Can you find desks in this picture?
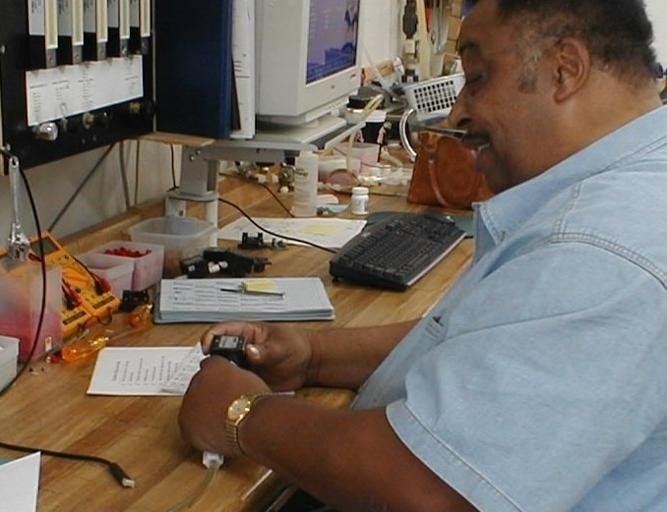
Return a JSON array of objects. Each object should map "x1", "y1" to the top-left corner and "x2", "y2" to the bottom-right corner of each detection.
[{"x1": 0, "y1": 164, "x2": 495, "y2": 512}]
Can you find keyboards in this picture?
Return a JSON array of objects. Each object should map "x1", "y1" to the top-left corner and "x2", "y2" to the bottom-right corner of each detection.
[{"x1": 329, "y1": 214, "x2": 468, "y2": 292}]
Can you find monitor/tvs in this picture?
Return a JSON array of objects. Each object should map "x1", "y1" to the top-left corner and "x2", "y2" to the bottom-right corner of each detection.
[{"x1": 254, "y1": 0, "x2": 362, "y2": 146}]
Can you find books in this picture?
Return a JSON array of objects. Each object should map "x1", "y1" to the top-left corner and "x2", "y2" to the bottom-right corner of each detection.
[
  {"x1": 153, "y1": 278, "x2": 335, "y2": 324},
  {"x1": 155, "y1": 0, "x2": 256, "y2": 139}
]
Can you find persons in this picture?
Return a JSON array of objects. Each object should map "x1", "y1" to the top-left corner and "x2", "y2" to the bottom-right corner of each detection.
[{"x1": 179, "y1": 1, "x2": 667, "y2": 511}]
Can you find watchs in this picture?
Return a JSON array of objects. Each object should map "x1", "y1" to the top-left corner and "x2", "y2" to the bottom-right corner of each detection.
[{"x1": 224, "y1": 390, "x2": 295, "y2": 458}]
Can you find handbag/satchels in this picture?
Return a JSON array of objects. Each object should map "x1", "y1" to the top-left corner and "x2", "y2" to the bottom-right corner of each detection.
[{"x1": 407, "y1": 125, "x2": 499, "y2": 210}]
